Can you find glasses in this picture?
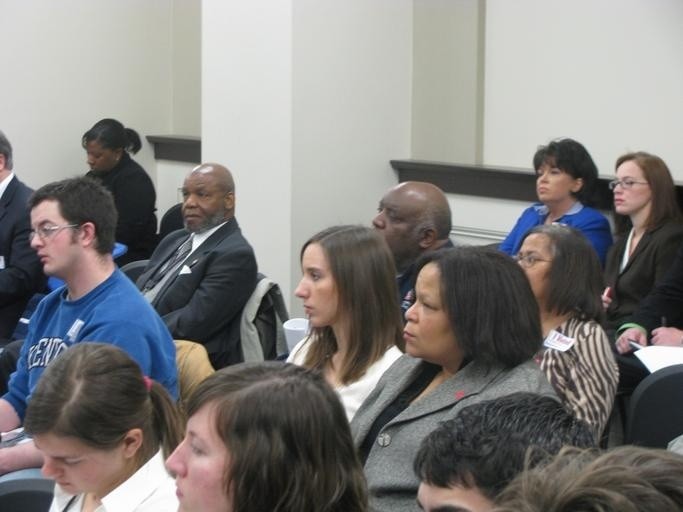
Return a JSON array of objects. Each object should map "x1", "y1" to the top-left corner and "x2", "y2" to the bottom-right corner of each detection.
[
  {"x1": 27, "y1": 224, "x2": 79, "y2": 242},
  {"x1": 512, "y1": 253, "x2": 551, "y2": 267},
  {"x1": 608, "y1": 179, "x2": 649, "y2": 190}
]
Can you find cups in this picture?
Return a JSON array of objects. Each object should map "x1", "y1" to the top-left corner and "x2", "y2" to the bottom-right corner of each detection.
[{"x1": 282, "y1": 317, "x2": 308, "y2": 354}]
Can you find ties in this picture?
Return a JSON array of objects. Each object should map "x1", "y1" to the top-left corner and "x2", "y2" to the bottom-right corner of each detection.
[{"x1": 143, "y1": 238, "x2": 192, "y2": 290}]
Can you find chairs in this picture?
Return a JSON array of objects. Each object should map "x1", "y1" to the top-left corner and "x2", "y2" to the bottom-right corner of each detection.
[
  {"x1": 620, "y1": 361, "x2": 682, "y2": 454},
  {"x1": 0, "y1": 465, "x2": 56, "y2": 512},
  {"x1": 113, "y1": 253, "x2": 282, "y2": 377},
  {"x1": 157, "y1": 201, "x2": 189, "y2": 240},
  {"x1": 585, "y1": 392, "x2": 626, "y2": 464}
]
